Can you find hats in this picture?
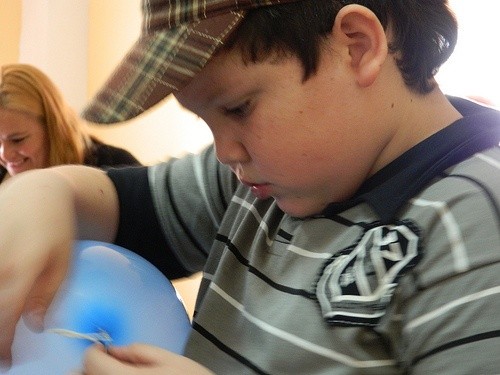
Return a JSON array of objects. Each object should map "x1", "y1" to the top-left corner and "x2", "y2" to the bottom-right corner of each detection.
[{"x1": 80, "y1": 0, "x2": 293, "y2": 124}]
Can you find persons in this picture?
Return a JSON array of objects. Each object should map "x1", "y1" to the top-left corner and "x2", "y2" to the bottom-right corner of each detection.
[
  {"x1": 0, "y1": 0, "x2": 500, "y2": 375},
  {"x1": 0, "y1": 63, "x2": 144, "y2": 185}
]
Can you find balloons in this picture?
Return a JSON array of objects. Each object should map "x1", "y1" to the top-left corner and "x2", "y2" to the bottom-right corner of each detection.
[{"x1": 0, "y1": 240, "x2": 191, "y2": 375}]
